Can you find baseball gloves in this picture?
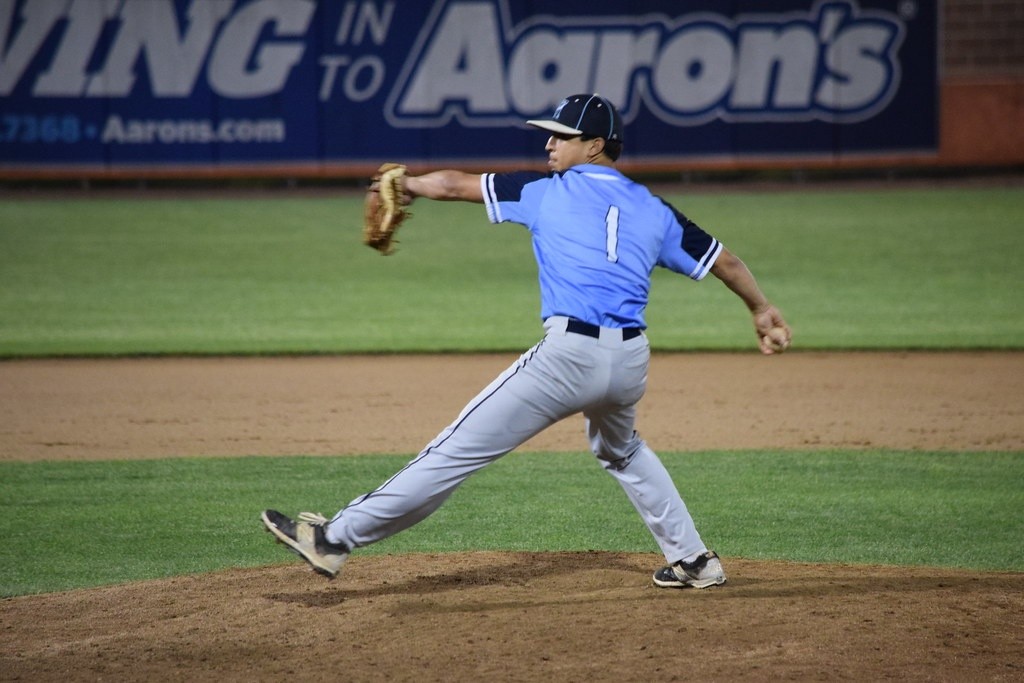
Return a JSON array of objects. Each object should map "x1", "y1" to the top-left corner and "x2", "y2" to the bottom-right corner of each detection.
[{"x1": 362, "y1": 161, "x2": 415, "y2": 257}]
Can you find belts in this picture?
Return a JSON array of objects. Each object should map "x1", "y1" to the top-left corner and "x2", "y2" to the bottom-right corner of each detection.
[{"x1": 565, "y1": 319, "x2": 641, "y2": 341}]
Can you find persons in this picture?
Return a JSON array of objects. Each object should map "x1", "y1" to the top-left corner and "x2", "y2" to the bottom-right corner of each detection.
[{"x1": 260, "y1": 92, "x2": 791, "y2": 589}]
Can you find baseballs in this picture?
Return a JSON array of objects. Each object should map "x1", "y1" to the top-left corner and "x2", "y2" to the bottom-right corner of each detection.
[{"x1": 763, "y1": 326, "x2": 789, "y2": 353}]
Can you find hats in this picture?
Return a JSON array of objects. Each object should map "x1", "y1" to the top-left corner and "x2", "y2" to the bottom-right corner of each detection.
[{"x1": 525, "y1": 93, "x2": 622, "y2": 143}]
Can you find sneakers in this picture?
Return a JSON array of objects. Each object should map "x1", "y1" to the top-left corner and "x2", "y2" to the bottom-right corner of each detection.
[
  {"x1": 653, "y1": 549, "x2": 727, "y2": 588},
  {"x1": 260, "y1": 508, "x2": 351, "y2": 578}
]
